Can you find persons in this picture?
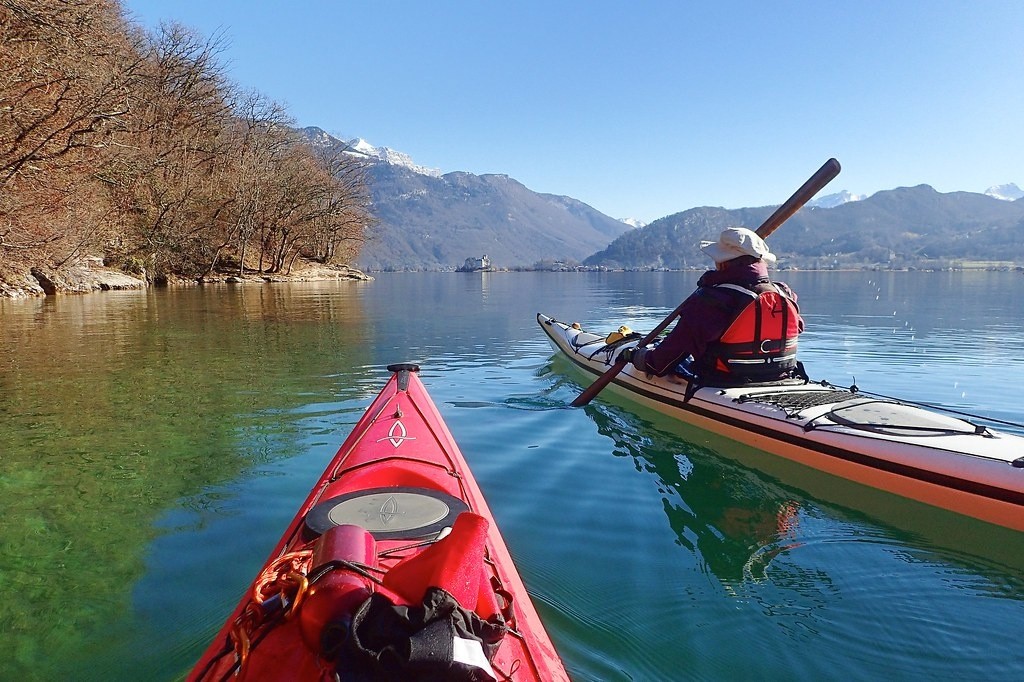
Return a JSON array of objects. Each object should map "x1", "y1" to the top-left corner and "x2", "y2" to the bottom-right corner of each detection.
[{"x1": 614, "y1": 227, "x2": 806, "y2": 388}]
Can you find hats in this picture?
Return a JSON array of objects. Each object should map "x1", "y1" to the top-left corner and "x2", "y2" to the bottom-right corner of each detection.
[{"x1": 700, "y1": 228, "x2": 777, "y2": 265}]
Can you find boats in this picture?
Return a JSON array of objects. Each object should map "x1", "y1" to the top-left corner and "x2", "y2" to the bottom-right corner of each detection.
[
  {"x1": 536, "y1": 312, "x2": 1024, "y2": 531},
  {"x1": 184, "y1": 364, "x2": 570, "y2": 682}
]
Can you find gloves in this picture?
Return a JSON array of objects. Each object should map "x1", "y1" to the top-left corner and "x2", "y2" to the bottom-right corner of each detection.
[{"x1": 615, "y1": 346, "x2": 640, "y2": 364}]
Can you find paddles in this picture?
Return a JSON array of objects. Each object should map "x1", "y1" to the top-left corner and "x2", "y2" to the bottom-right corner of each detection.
[{"x1": 569, "y1": 157, "x2": 843, "y2": 408}]
